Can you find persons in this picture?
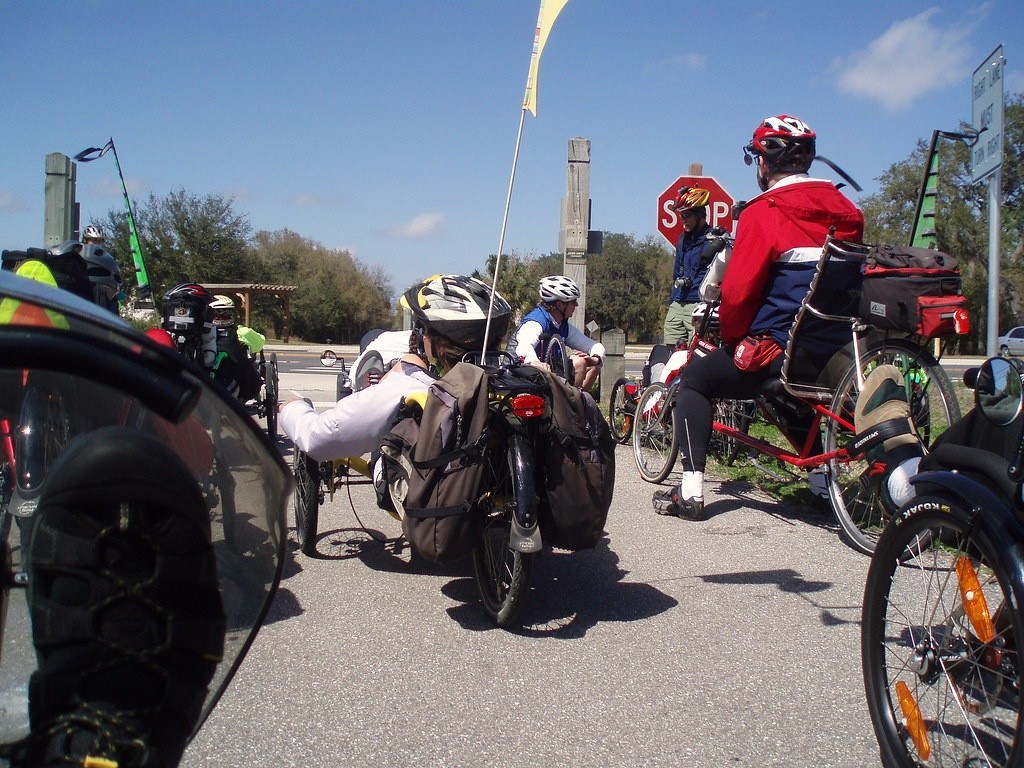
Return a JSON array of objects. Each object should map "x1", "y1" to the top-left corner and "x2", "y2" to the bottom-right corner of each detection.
[
  {"x1": 21, "y1": 226, "x2": 261, "y2": 542},
  {"x1": 0, "y1": 429, "x2": 226, "y2": 768},
  {"x1": 654, "y1": 116, "x2": 866, "y2": 522},
  {"x1": 854, "y1": 364, "x2": 1024, "y2": 548},
  {"x1": 278, "y1": 274, "x2": 510, "y2": 525},
  {"x1": 664, "y1": 187, "x2": 725, "y2": 360},
  {"x1": 503, "y1": 275, "x2": 606, "y2": 399}
]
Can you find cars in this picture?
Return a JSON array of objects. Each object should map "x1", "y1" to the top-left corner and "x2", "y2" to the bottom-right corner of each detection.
[{"x1": 997, "y1": 326, "x2": 1024, "y2": 357}]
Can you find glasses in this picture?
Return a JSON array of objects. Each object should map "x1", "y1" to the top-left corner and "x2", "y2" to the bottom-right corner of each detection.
[
  {"x1": 681, "y1": 211, "x2": 698, "y2": 219},
  {"x1": 754, "y1": 154, "x2": 760, "y2": 165},
  {"x1": 89, "y1": 237, "x2": 103, "y2": 245},
  {"x1": 566, "y1": 298, "x2": 577, "y2": 304}
]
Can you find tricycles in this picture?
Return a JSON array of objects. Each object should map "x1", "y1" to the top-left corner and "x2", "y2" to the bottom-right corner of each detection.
[
  {"x1": 0, "y1": 271, "x2": 298, "y2": 768},
  {"x1": 610, "y1": 225, "x2": 963, "y2": 563},
  {"x1": 861, "y1": 358, "x2": 1024, "y2": 768},
  {"x1": 292, "y1": 350, "x2": 550, "y2": 628}
]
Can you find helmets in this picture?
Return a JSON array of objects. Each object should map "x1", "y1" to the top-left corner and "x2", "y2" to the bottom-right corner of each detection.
[
  {"x1": 673, "y1": 186, "x2": 710, "y2": 211},
  {"x1": 539, "y1": 276, "x2": 581, "y2": 303},
  {"x1": 398, "y1": 273, "x2": 513, "y2": 352},
  {"x1": 162, "y1": 283, "x2": 215, "y2": 305},
  {"x1": 83, "y1": 224, "x2": 106, "y2": 240},
  {"x1": 209, "y1": 295, "x2": 234, "y2": 311},
  {"x1": 748, "y1": 114, "x2": 816, "y2": 166}
]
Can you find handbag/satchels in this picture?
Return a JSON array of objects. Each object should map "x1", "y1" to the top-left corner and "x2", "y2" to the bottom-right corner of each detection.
[
  {"x1": 400, "y1": 358, "x2": 616, "y2": 558},
  {"x1": 859, "y1": 245, "x2": 960, "y2": 335}
]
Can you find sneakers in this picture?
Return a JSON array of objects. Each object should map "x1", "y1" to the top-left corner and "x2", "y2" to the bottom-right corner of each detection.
[
  {"x1": 21, "y1": 427, "x2": 226, "y2": 768},
  {"x1": 653, "y1": 483, "x2": 703, "y2": 521},
  {"x1": 373, "y1": 456, "x2": 392, "y2": 510},
  {"x1": 844, "y1": 364, "x2": 929, "y2": 517}
]
